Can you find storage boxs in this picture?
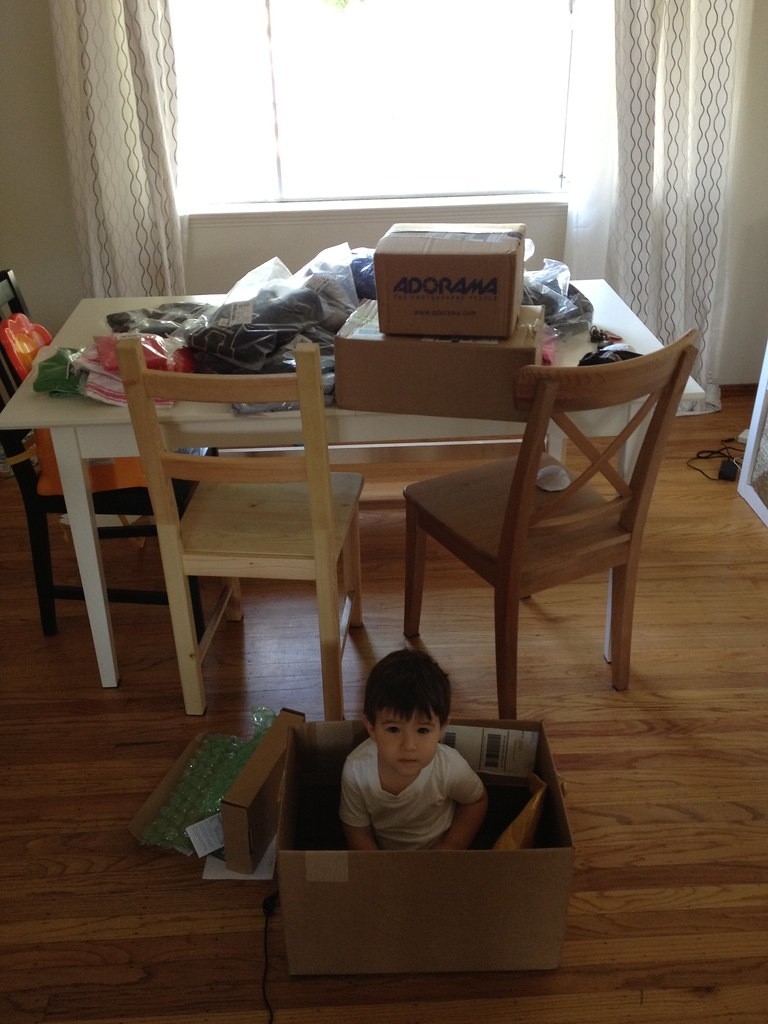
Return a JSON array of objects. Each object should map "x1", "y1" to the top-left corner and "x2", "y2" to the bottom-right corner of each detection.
[
  {"x1": 124, "y1": 708, "x2": 303, "y2": 876},
  {"x1": 371, "y1": 222, "x2": 528, "y2": 337},
  {"x1": 333, "y1": 295, "x2": 550, "y2": 423},
  {"x1": 273, "y1": 712, "x2": 580, "y2": 979}
]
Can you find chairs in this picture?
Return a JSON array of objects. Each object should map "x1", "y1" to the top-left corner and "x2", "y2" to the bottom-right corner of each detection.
[
  {"x1": 401, "y1": 328, "x2": 694, "y2": 723},
  {"x1": 116, "y1": 333, "x2": 362, "y2": 718},
  {"x1": 0, "y1": 269, "x2": 216, "y2": 636}
]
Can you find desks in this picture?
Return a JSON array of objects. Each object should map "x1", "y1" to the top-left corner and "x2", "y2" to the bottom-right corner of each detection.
[
  {"x1": 1, "y1": 279, "x2": 706, "y2": 688},
  {"x1": 0, "y1": 277, "x2": 708, "y2": 690}
]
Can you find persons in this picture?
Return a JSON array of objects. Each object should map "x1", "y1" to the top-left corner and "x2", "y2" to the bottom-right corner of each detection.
[{"x1": 335, "y1": 649, "x2": 489, "y2": 850}]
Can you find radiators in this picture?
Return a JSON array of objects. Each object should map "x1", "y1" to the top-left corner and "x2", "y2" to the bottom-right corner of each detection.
[{"x1": 183, "y1": 199, "x2": 568, "y2": 304}]
